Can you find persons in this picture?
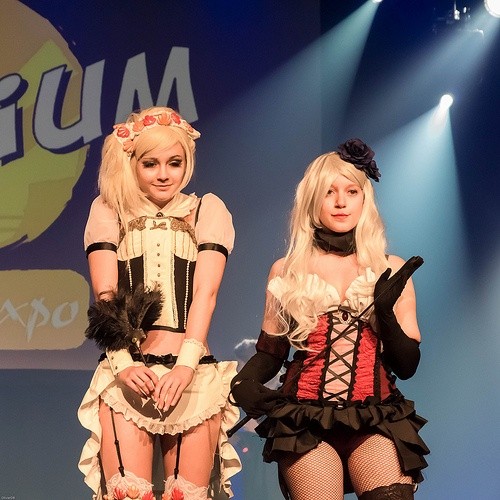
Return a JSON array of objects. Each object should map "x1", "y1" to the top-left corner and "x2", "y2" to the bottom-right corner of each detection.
[
  {"x1": 78, "y1": 106, "x2": 242, "y2": 500},
  {"x1": 227, "y1": 138, "x2": 430, "y2": 500}
]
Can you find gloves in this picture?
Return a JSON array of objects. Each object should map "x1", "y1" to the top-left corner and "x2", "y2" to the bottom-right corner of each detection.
[
  {"x1": 372, "y1": 255, "x2": 421, "y2": 382},
  {"x1": 229, "y1": 330, "x2": 287, "y2": 420}
]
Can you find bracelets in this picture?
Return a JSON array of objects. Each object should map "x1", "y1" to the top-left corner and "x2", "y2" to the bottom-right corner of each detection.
[
  {"x1": 175, "y1": 339, "x2": 206, "y2": 370},
  {"x1": 104, "y1": 347, "x2": 135, "y2": 374}
]
